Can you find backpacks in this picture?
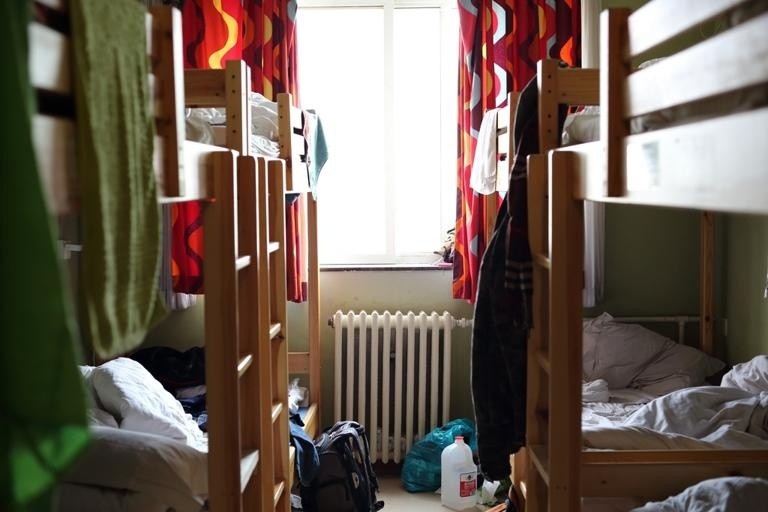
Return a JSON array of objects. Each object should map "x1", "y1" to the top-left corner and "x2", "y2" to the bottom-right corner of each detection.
[{"x1": 301, "y1": 421, "x2": 384, "y2": 512}]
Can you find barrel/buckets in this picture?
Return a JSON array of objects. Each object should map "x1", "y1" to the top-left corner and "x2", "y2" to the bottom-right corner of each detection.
[{"x1": 434, "y1": 431, "x2": 483, "y2": 508}]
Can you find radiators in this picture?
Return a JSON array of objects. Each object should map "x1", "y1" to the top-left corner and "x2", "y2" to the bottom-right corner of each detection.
[{"x1": 330, "y1": 308, "x2": 472, "y2": 468}]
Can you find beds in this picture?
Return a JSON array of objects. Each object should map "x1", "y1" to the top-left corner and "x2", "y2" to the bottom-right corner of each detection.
[
  {"x1": 527, "y1": 1, "x2": 767, "y2": 512},
  {"x1": 475, "y1": 57, "x2": 767, "y2": 512},
  {"x1": 0, "y1": 0, "x2": 265, "y2": 512},
  {"x1": 54, "y1": 56, "x2": 329, "y2": 512}
]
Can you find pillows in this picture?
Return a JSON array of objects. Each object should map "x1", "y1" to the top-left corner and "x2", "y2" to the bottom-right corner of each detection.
[
  {"x1": 579, "y1": 309, "x2": 615, "y2": 379},
  {"x1": 581, "y1": 313, "x2": 724, "y2": 401},
  {"x1": 59, "y1": 423, "x2": 208, "y2": 497}
]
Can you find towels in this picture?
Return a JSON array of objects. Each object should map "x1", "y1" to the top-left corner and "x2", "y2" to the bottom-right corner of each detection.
[
  {"x1": 469, "y1": 107, "x2": 501, "y2": 195},
  {"x1": 301, "y1": 107, "x2": 329, "y2": 201}
]
